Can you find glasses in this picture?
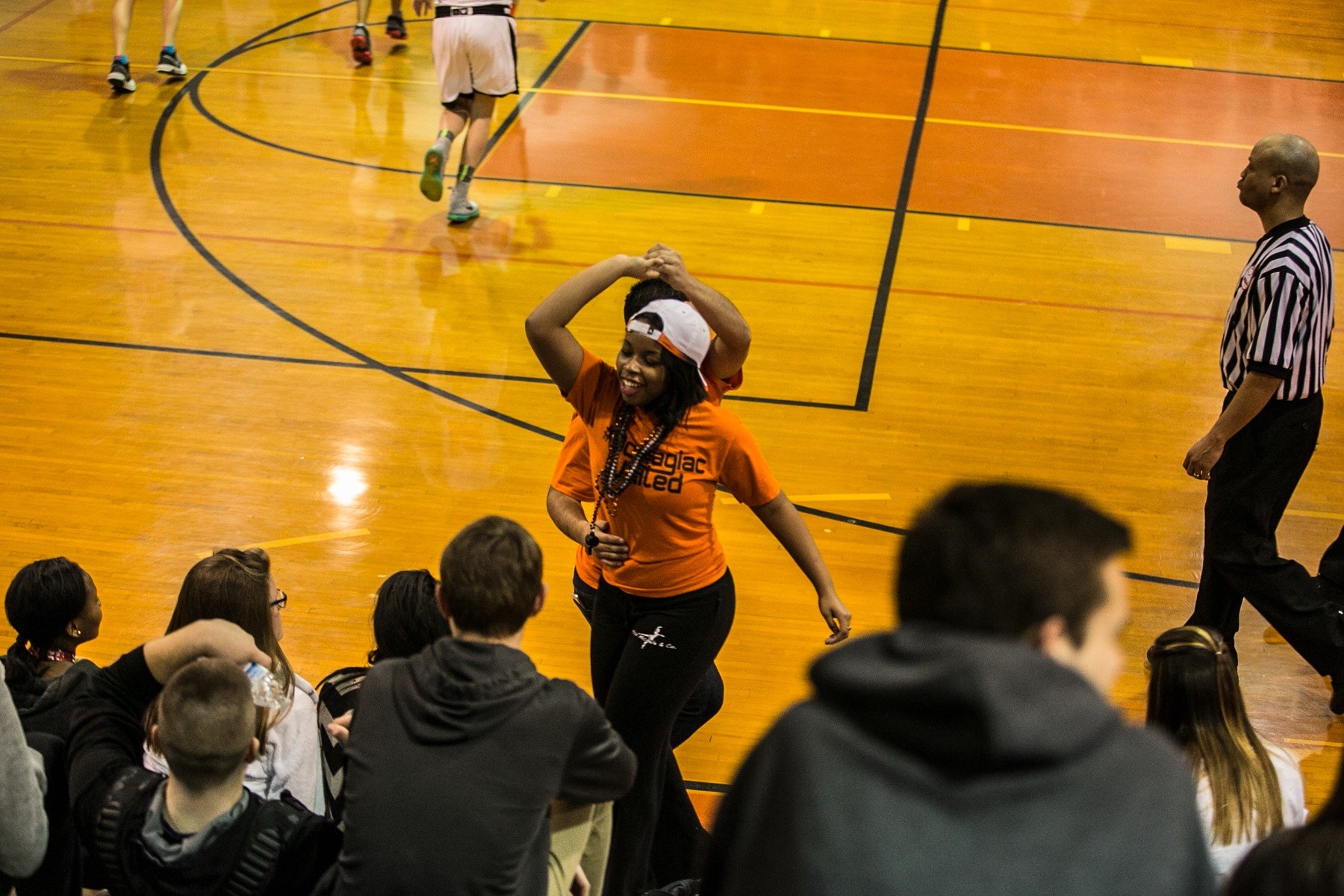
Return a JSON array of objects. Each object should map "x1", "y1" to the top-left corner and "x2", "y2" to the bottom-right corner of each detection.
[{"x1": 270, "y1": 588, "x2": 287, "y2": 609}]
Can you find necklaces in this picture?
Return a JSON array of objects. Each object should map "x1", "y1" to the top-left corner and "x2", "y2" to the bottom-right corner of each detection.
[{"x1": 584, "y1": 392, "x2": 679, "y2": 555}]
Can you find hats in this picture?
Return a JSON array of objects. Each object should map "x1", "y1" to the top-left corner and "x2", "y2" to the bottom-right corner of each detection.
[{"x1": 627, "y1": 298, "x2": 713, "y2": 387}]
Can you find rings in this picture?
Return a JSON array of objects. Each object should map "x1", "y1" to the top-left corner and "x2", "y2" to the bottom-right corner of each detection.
[{"x1": 847, "y1": 626, "x2": 851, "y2": 630}]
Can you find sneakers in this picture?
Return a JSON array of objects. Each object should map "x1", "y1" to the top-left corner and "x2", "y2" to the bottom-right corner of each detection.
[
  {"x1": 105, "y1": 60, "x2": 137, "y2": 92},
  {"x1": 447, "y1": 197, "x2": 479, "y2": 222},
  {"x1": 420, "y1": 140, "x2": 449, "y2": 201},
  {"x1": 155, "y1": 51, "x2": 188, "y2": 75}
]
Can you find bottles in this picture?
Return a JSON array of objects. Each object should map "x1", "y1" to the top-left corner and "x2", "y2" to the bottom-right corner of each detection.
[{"x1": 241, "y1": 661, "x2": 291, "y2": 711}]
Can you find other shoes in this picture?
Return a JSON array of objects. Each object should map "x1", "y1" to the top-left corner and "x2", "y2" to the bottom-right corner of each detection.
[
  {"x1": 386, "y1": 15, "x2": 408, "y2": 40},
  {"x1": 1328, "y1": 611, "x2": 1344, "y2": 715},
  {"x1": 351, "y1": 23, "x2": 373, "y2": 65},
  {"x1": 1264, "y1": 625, "x2": 1288, "y2": 645}
]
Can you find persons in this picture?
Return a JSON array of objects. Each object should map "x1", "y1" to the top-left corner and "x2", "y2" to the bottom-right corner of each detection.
[
  {"x1": 1146, "y1": 134, "x2": 1344, "y2": 714},
  {"x1": 1226, "y1": 525, "x2": 1344, "y2": 896},
  {"x1": 0, "y1": 514, "x2": 634, "y2": 896},
  {"x1": 353, "y1": 0, "x2": 553, "y2": 219},
  {"x1": 1142, "y1": 625, "x2": 1307, "y2": 888},
  {"x1": 107, "y1": 0, "x2": 188, "y2": 94},
  {"x1": 693, "y1": 476, "x2": 1217, "y2": 896},
  {"x1": 544, "y1": 242, "x2": 751, "y2": 754},
  {"x1": 525, "y1": 252, "x2": 852, "y2": 896}
]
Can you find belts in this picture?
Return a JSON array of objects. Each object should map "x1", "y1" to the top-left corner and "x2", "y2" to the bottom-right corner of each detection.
[{"x1": 435, "y1": 6, "x2": 512, "y2": 16}]
